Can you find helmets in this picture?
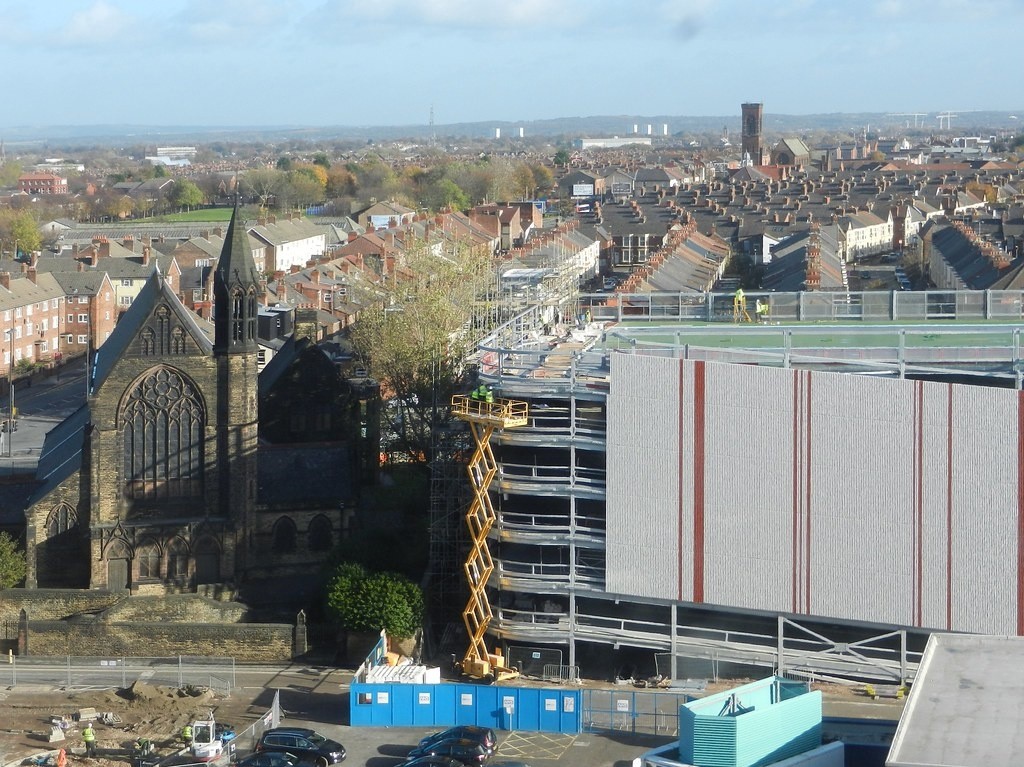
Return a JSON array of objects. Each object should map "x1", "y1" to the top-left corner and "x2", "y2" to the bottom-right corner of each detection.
[{"x1": 88, "y1": 723, "x2": 92, "y2": 726}]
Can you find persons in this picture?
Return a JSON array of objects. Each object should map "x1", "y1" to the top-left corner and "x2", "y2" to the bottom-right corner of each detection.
[
  {"x1": 537, "y1": 314, "x2": 549, "y2": 336},
  {"x1": 136, "y1": 737, "x2": 150, "y2": 755},
  {"x1": 754, "y1": 299, "x2": 762, "y2": 323},
  {"x1": 182, "y1": 723, "x2": 192, "y2": 747},
  {"x1": 82, "y1": 722, "x2": 97, "y2": 758},
  {"x1": 736, "y1": 288, "x2": 744, "y2": 309},
  {"x1": 585, "y1": 310, "x2": 591, "y2": 324},
  {"x1": 472, "y1": 383, "x2": 494, "y2": 414}
]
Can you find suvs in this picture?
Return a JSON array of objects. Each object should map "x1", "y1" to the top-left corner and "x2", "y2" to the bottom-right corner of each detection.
[
  {"x1": 407, "y1": 736, "x2": 491, "y2": 767},
  {"x1": 255, "y1": 726, "x2": 347, "y2": 765},
  {"x1": 417, "y1": 725, "x2": 499, "y2": 757}
]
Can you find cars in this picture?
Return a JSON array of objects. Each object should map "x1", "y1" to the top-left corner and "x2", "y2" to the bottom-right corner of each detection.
[
  {"x1": 386, "y1": 392, "x2": 419, "y2": 410},
  {"x1": 231, "y1": 747, "x2": 328, "y2": 767},
  {"x1": 392, "y1": 754, "x2": 465, "y2": 767},
  {"x1": 379, "y1": 435, "x2": 472, "y2": 469}
]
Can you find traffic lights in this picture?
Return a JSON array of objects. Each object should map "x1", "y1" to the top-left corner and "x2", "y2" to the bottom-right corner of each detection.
[
  {"x1": 2, "y1": 420, "x2": 9, "y2": 433},
  {"x1": 13, "y1": 420, "x2": 18, "y2": 431}
]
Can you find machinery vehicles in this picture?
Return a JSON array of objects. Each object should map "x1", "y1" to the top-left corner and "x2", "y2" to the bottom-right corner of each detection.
[
  {"x1": 449, "y1": 393, "x2": 530, "y2": 684},
  {"x1": 192, "y1": 710, "x2": 225, "y2": 763}
]
